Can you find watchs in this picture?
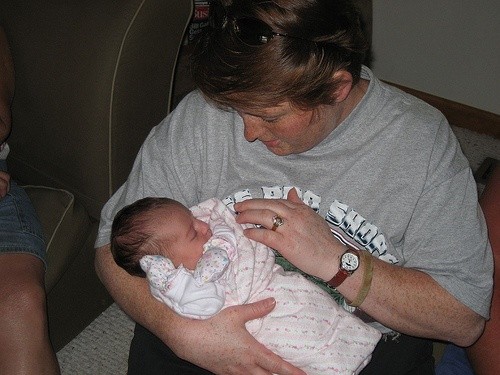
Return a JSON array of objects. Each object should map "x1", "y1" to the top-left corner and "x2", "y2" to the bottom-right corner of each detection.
[{"x1": 325, "y1": 243, "x2": 361, "y2": 291}]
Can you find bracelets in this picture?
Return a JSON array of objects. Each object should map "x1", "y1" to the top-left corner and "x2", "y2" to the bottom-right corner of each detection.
[{"x1": 345, "y1": 249, "x2": 374, "y2": 308}]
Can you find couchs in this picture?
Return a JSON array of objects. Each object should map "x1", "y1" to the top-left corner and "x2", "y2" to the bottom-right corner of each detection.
[{"x1": 0, "y1": 0, "x2": 194, "y2": 353}]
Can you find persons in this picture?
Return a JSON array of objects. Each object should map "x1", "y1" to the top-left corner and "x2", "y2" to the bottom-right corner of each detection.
[
  {"x1": 92, "y1": 1, "x2": 493, "y2": 375},
  {"x1": 1, "y1": 39, "x2": 60, "y2": 375}
]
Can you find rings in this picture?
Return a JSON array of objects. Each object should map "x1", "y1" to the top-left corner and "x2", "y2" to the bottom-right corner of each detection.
[{"x1": 271, "y1": 214, "x2": 283, "y2": 230}]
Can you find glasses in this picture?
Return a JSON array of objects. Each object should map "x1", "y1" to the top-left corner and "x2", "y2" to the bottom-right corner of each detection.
[{"x1": 209, "y1": 2, "x2": 297, "y2": 45}]
